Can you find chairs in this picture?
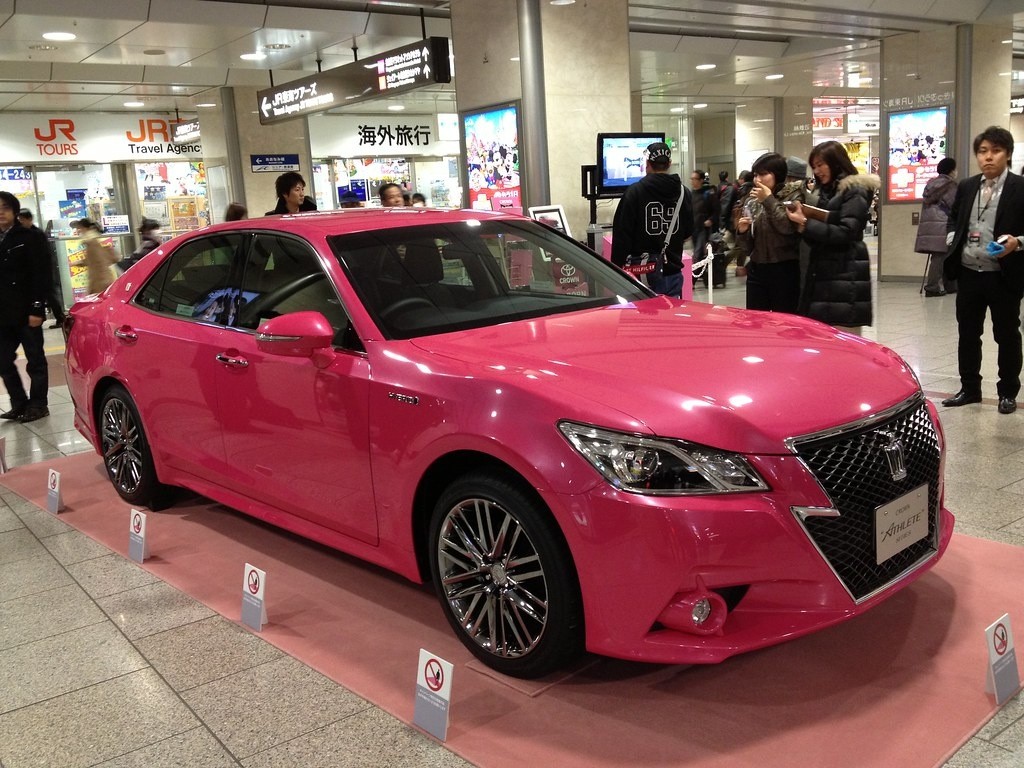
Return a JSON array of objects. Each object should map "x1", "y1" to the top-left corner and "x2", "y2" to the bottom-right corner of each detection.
[{"x1": 405, "y1": 241, "x2": 455, "y2": 311}]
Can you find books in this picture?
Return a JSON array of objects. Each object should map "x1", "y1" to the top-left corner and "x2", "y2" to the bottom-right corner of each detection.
[{"x1": 801, "y1": 204, "x2": 829, "y2": 223}]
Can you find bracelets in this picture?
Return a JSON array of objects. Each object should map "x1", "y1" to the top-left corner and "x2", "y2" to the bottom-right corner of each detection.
[{"x1": 798, "y1": 218, "x2": 807, "y2": 232}]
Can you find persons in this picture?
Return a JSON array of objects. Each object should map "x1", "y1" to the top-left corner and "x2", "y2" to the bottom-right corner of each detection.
[
  {"x1": 339, "y1": 190, "x2": 360, "y2": 208},
  {"x1": 17, "y1": 208, "x2": 66, "y2": 329},
  {"x1": 714, "y1": 171, "x2": 753, "y2": 277},
  {"x1": 785, "y1": 140, "x2": 882, "y2": 338},
  {"x1": 215, "y1": 203, "x2": 249, "y2": 264},
  {"x1": 610, "y1": 143, "x2": 694, "y2": 300},
  {"x1": 733, "y1": 153, "x2": 806, "y2": 313},
  {"x1": 0, "y1": 190, "x2": 53, "y2": 423},
  {"x1": 689, "y1": 169, "x2": 708, "y2": 290},
  {"x1": 116, "y1": 219, "x2": 166, "y2": 271},
  {"x1": 941, "y1": 127, "x2": 1024, "y2": 414},
  {"x1": 254, "y1": 171, "x2": 317, "y2": 271},
  {"x1": 378, "y1": 183, "x2": 445, "y2": 285},
  {"x1": 639, "y1": 149, "x2": 650, "y2": 177},
  {"x1": 914, "y1": 157, "x2": 958, "y2": 298}
]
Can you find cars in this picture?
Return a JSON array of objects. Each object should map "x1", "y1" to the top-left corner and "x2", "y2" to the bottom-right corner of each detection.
[{"x1": 61, "y1": 207, "x2": 955, "y2": 680}]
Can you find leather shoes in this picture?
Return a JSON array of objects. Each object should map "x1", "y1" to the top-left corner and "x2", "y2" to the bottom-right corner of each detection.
[
  {"x1": 998, "y1": 396, "x2": 1017, "y2": 414},
  {"x1": 941, "y1": 389, "x2": 982, "y2": 406}
]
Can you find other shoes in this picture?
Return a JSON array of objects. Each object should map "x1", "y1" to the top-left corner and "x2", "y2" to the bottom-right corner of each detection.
[
  {"x1": 713, "y1": 283, "x2": 724, "y2": 289},
  {"x1": 736, "y1": 266, "x2": 745, "y2": 276},
  {"x1": 16, "y1": 406, "x2": 50, "y2": 423},
  {"x1": 946, "y1": 287, "x2": 957, "y2": 294},
  {"x1": 924, "y1": 288, "x2": 947, "y2": 297},
  {"x1": 0, "y1": 407, "x2": 27, "y2": 419},
  {"x1": 49, "y1": 317, "x2": 66, "y2": 329}
]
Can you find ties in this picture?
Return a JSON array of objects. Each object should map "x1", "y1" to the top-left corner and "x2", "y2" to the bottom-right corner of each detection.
[{"x1": 980, "y1": 178, "x2": 995, "y2": 204}]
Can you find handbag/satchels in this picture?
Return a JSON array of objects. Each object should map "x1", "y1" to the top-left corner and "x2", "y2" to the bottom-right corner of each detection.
[{"x1": 623, "y1": 253, "x2": 664, "y2": 287}]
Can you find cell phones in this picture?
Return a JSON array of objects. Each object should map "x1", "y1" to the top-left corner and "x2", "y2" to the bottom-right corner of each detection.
[
  {"x1": 783, "y1": 201, "x2": 795, "y2": 211},
  {"x1": 997, "y1": 236, "x2": 1008, "y2": 245}
]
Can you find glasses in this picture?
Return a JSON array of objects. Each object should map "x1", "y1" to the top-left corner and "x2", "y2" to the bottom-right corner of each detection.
[{"x1": 688, "y1": 177, "x2": 699, "y2": 182}]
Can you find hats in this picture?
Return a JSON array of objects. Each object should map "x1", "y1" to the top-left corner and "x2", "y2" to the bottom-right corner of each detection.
[
  {"x1": 937, "y1": 158, "x2": 956, "y2": 175},
  {"x1": 784, "y1": 155, "x2": 808, "y2": 179}
]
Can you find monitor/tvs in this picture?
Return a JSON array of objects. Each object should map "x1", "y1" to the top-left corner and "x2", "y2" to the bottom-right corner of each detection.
[{"x1": 596, "y1": 132, "x2": 666, "y2": 193}]
